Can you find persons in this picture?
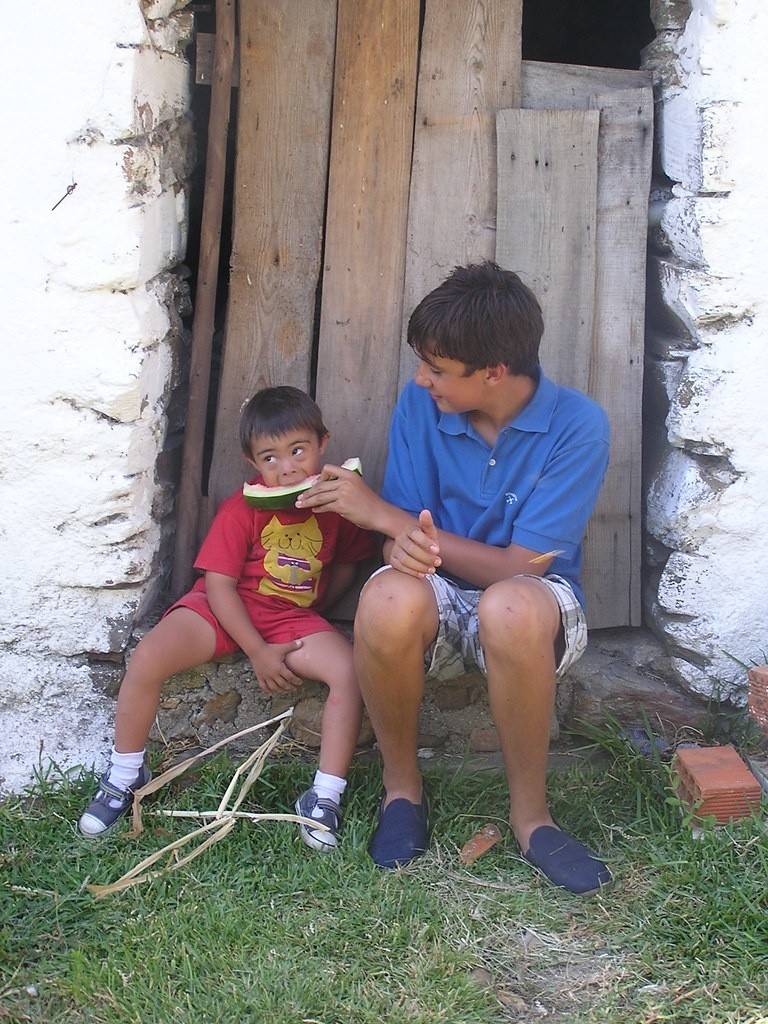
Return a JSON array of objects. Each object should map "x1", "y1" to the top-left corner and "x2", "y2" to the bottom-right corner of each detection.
[
  {"x1": 79, "y1": 384, "x2": 363, "y2": 853},
  {"x1": 295, "y1": 256, "x2": 614, "y2": 897}
]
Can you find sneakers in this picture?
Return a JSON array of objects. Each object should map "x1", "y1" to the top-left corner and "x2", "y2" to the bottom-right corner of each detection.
[
  {"x1": 79, "y1": 764, "x2": 152, "y2": 837},
  {"x1": 294, "y1": 787, "x2": 342, "y2": 852}
]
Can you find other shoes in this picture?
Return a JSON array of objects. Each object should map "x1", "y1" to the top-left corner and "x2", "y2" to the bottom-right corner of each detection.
[
  {"x1": 368, "y1": 785, "x2": 430, "y2": 870},
  {"x1": 508, "y1": 814, "x2": 614, "y2": 896}
]
